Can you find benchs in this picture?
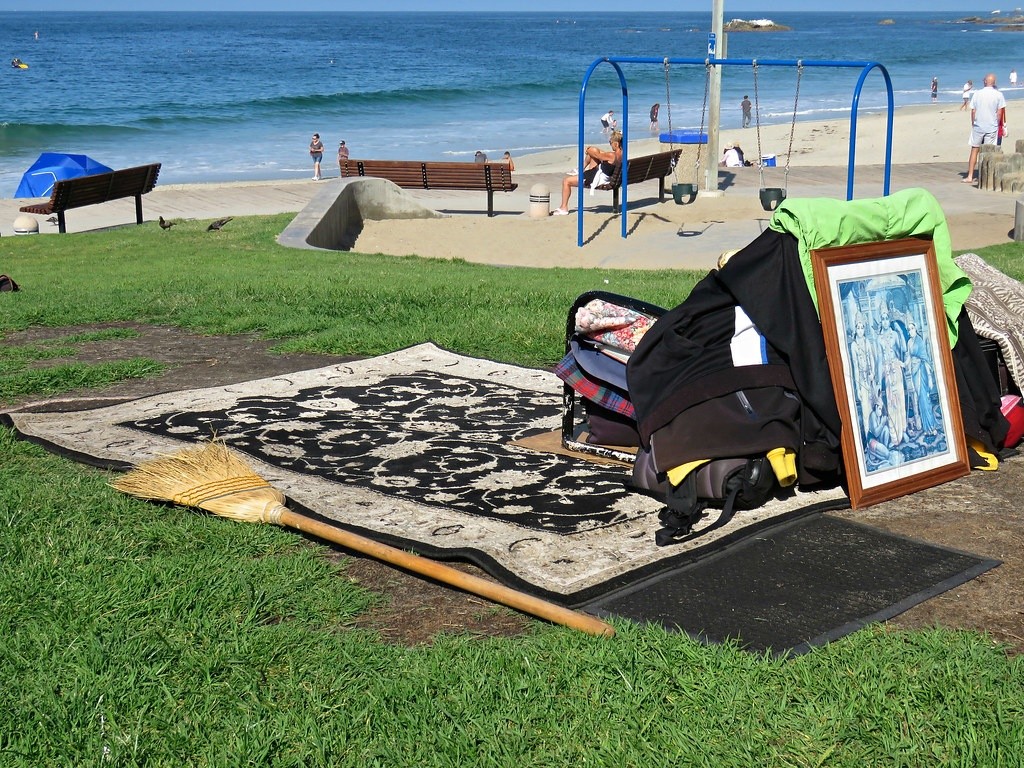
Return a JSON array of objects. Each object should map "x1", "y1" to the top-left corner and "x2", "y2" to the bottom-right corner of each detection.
[
  {"x1": 596, "y1": 149, "x2": 683, "y2": 214},
  {"x1": 339, "y1": 158, "x2": 519, "y2": 217},
  {"x1": 19, "y1": 162, "x2": 162, "y2": 233}
]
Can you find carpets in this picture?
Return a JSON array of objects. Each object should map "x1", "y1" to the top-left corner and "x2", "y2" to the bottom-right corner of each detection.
[
  {"x1": 0, "y1": 338, "x2": 849, "y2": 611},
  {"x1": 585, "y1": 512, "x2": 1002, "y2": 657}
]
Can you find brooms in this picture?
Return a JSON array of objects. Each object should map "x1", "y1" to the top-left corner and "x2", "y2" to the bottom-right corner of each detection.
[{"x1": 98, "y1": 425, "x2": 616, "y2": 640}]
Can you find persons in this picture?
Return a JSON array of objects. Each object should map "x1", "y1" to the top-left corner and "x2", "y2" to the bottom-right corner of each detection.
[
  {"x1": 719, "y1": 142, "x2": 744, "y2": 167},
  {"x1": 35, "y1": 31, "x2": 39, "y2": 40},
  {"x1": 337, "y1": 140, "x2": 349, "y2": 178},
  {"x1": 503, "y1": 151, "x2": 514, "y2": 171},
  {"x1": 551, "y1": 133, "x2": 624, "y2": 214},
  {"x1": 960, "y1": 80, "x2": 973, "y2": 111},
  {"x1": 309, "y1": 134, "x2": 324, "y2": 182},
  {"x1": 741, "y1": 95, "x2": 752, "y2": 128},
  {"x1": 475, "y1": 151, "x2": 487, "y2": 164},
  {"x1": 931, "y1": 77, "x2": 938, "y2": 101},
  {"x1": 961, "y1": 73, "x2": 1008, "y2": 183},
  {"x1": 649, "y1": 104, "x2": 661, "y2": 130},
  {"x1": 600, "y1": 111, "x2": 617, "y2": 133},
  {"x1": 1009, "y1": 69, "x2": 1016, "y2": 87}
]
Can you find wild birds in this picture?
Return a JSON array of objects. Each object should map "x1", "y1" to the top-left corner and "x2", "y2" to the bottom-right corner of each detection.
[
  {"x1": 46, "y1": 217, "x2": 59, "y2": 225},
  {"x1": 158, "y1": 215, "x2": 176, "y2": 231},
  {"x1": 206, "y1": 217, "x2": 233, "y2": 231}
]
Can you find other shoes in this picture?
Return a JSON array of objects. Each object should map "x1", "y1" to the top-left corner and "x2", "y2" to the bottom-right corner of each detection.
[
  {"x1": 312, "y1": 177, "x2": 318, "y2": 181},
  {"x1": 318, "y1": 177, "x2": 320, "y2": 180},
  {"x1": 962, "y1": 178, "x2": 972, "y2": 183},
  {"x1": 549, "y1": 208, "x2": 569, "y2": 215},
  {"x1": 566, "y1": 168, "x2": 578, "y2": 175}
]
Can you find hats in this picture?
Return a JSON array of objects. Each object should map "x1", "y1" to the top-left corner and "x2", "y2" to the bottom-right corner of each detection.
[
  {"x1": 475, "y1": 151, "x2": 481, "y2": 156},
  {"x1": 340, "y1": 141, "x2": 345, "y2": 144},
  {"x1": 724, "y1": 142, "x2": 734, "y2": 149},
  {"x1": 733, "y1": 142, "x2": 740, "y2": 148},
  {"x1": 744, "y1": 95, "x2": 748, "y2": 99}
]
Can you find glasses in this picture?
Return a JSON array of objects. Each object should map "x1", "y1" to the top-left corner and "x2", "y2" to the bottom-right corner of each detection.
[
  {"x1": 610, "y1": 141, "x2": 613, "y2": 145},
  {"x1": 312, "y1": 137, "x2": 318, "y2": 141}
]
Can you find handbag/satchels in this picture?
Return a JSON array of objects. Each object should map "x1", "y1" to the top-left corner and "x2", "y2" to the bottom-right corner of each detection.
[
  {"x1": 1002, "y1": 123, "x2": 1008, "y2": 137},
  {"x1": 567, "y1": 290, "x2": 669, "y2": 393},
  {"x1": 623, "y1": 445, "x2": 776, "y2": 546},
  {"x1": 0, "y1": 275, "x2": 18, "y2": 292},
  {"x1": 580, "y1": 396, "x2": 643, "y2": 444}
]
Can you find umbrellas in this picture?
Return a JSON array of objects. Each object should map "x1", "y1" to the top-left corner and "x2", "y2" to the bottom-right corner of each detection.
[{"x1": 15, "y1": 152, "x2": 116, "y2": 199}]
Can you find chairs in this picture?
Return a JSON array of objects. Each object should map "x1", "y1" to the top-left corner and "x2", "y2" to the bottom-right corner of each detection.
[{"x1": 560, "y1": 291, "x2": 671, "y2": 464}]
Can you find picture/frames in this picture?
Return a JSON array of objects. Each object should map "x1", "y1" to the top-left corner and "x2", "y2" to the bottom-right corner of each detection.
[{"x1": 810, "y1": 237, "x2": 972, "y2": 510}]
